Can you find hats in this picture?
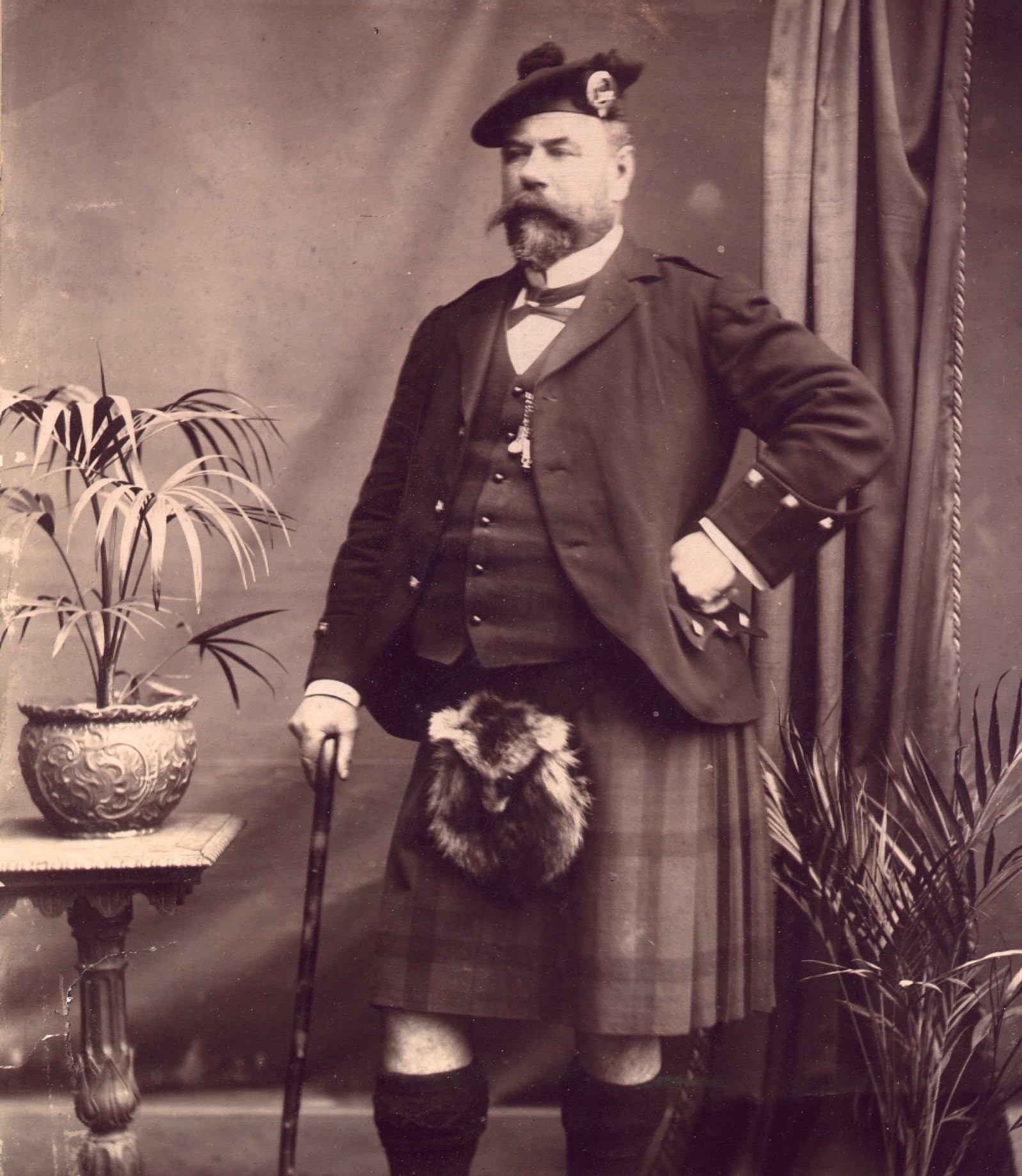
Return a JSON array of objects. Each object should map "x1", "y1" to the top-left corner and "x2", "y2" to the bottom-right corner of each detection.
[{"x1": 469, "y1": 41, "x2": 646, "y2": 150}]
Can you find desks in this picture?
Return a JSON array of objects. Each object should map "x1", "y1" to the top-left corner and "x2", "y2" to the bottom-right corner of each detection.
[{"x1": 0, "y1": 812, "x2": 250, "y2": 1176}]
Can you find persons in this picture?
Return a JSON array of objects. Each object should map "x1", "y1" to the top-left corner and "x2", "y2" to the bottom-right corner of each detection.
[{"x1": 286, "y1": 40, "x2": 897, "y2": 1176}]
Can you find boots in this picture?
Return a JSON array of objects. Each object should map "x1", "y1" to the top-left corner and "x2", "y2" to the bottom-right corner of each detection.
[
  {"x1": 559, "y1": 1058, "x2": 672, "y2": 1176},
  {"x1": 371, "y1": 1055, "x2": 491, "y2": 1176}
]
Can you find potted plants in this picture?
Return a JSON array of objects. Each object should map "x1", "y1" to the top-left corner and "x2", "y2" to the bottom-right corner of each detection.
[{"x1": 0, "y1": 348, "x2": 303, "y2": 843}]
